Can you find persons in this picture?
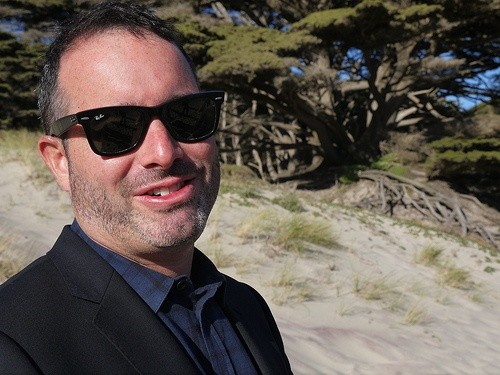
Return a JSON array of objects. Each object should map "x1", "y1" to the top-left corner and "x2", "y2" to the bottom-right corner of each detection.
[{"x1": 0, "y1": 5, "x2": 294, "y2": 375}]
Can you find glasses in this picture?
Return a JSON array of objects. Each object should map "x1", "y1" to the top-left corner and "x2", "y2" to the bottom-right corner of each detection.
[{"x1": 45, "y1": 91, "x2": 225, "y2": 156}]
